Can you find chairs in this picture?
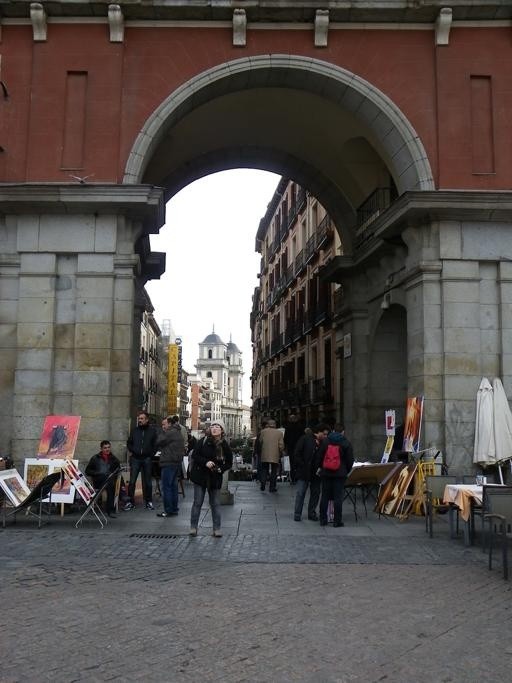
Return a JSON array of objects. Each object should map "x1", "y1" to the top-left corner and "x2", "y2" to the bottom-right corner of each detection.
[
  {"x1": 462, "y1": 475, "x2": 497, "y2": 545},
  {"x1": 470, "y1": 484, "x2": 512, "y2": 545},
  {"x1": 425, "y1": 475, "x2": 460, "y2": 539},
  {"x1": 485, "y1": 493, "x2": 512, "y2": 581}
]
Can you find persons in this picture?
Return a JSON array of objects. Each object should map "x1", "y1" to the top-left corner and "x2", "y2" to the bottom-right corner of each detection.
[
  {"x1": 161, "y1": 418, "x2": 169, "y2": 432},
  {"x1": 294, "y1": 423, "x2": 331, "y2": 522},
  {"x1": 173, "y1": 415, "x2": 189, "y2": 479},
  {"x1": 188, "y1": 448, "x2": 194, "y2": 485},
  {"x1": 259, "y1": 420, "x2": 284, "y2": 493},
  {"x1": 316, "y1": 424, "x2": 354, "y2": 527},
  {"x1": 120, "y1": 410, "x2": 158, "y2": 511},
  {"x1": 182, "y1": 433, "x2": 200, "y2": 479},
  {"x1": 283, "y1": 415, "x2": 305, "y2": 487},
  {"x1": 187, "y1": 419, "x2": 232, "y2": 537},
  {"x1": 156, "y1": 417, "x2": 187, "y2": 517},
  {"x1": 305, "y1": 428, "x2": 312, "y2": 435},
  {"x1": 85, "y1": 440, "x2": 131, "y2": 518},
  {"x1": 228, "y1": 462, "x2": 257, "y2": 481}
]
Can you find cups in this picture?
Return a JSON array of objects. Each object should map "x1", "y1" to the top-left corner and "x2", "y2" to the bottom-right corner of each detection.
[{"x1": 475, "y1": 476, "x2": 484, "y2": 486}]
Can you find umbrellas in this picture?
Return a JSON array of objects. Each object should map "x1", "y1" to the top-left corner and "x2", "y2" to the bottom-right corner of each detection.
[
  {"x1": 473, "y1": 377, "x2": 504, "y2": 484},
  {"x1": 489, "y1": 377, "x2": 512, "y2": 470}
]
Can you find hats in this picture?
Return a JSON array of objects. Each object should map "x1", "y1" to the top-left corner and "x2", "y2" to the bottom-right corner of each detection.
[{"x1": 211, "y1": 417, "x2": 226, "y2": 432}]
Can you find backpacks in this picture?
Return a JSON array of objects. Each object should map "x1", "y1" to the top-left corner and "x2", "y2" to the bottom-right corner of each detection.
[{"x1": 324, "y1": 445, "x2": 342, "y2": 470}]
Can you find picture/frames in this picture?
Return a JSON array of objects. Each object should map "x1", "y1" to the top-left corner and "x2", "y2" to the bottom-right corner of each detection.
[
  {"x1": 19, "y1": 473, "x2": 60, "y2": 506},
  {"x1": 101, "y1": 472, "x2": 143, "y2": 509},
  {"x1": 384, "y1": 435, "x2": 395, "y2": 455},
  {"x1": 386, "y1": 410, "x2": 395, "y2": 435},
  {"x1": 0, "y1": 468, "x2": 31, "y2": 506},
  {"x1": 51, "y1": 458, "x2": 78, "y2": 504},
  {"x1": 152, "y1": 451, "x2": 188, "y2": 479},
  {"x1": 380, "y1": 454, "x2": 390, "y2": 463},
  {"x1": 62, "y1": 461, "x2": 96, "y2": 506},
  {"x1": 345, "y1": 462, "x2": 402, "y2": 485},
  {"x1": 25, "y1": 458, "x2": 51, "y2": 502},
  {"x1": 37, "y1": 415, "x2": 81, "y2": 459},
  {"x1": 375, "y1": 463, "x2": 418, "y2": 516},
  {"x1": 404, "y1": 396, "x2": 423, "y2": 453}
]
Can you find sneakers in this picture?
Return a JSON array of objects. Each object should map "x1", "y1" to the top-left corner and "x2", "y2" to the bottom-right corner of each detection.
[
  {"x1": 122, "y1": 502, "x2": 135, "y2": 512},
  {"x1": 308, "y1": 515, "x2": 318, "y2": 521},
  {"x1": 294, "y1": 516, "x2": 302, "y2": 521},
  {"x1": 158, "y1": 512, "x2": 172, "y2": 518},
  {"x1": 334, "y1": 520, "x2": 344, "y2": 528},
  {"x1": 144, "y1": 501, "x2": 154, "y2": 510},
  {"x1": 189, "y1": 528, "x2": 199, "y2": 535},
  {"x1": 320, "y1": 520, "x2": 328, "y2": 527},
  {"x1": 214, "y1": 526, "x2": 223, "y2": 537}
]
